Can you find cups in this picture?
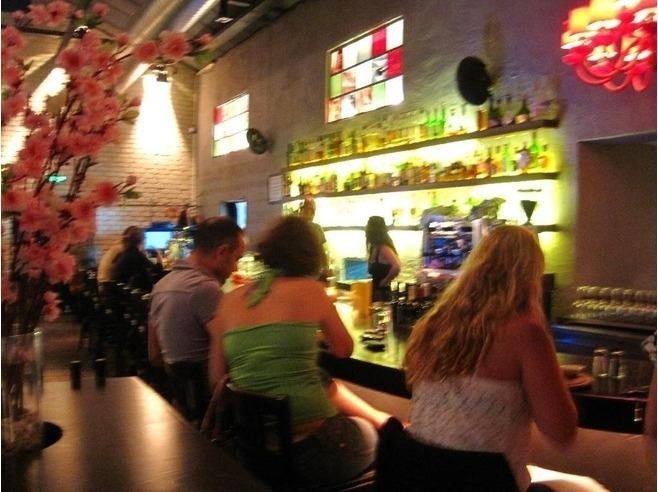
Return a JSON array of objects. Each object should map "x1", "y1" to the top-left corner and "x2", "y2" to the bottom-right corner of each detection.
[{"x1": 368, "y1": 304, "x2": 392, "y2": 330}]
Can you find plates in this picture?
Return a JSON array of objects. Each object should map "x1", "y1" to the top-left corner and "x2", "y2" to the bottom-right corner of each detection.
[
  {"x1": 0, "y1": 419, "x2": 63, "y2": 462},
  {"x1": 560, "y1": 374, "x2": 594, "y2": 389}
]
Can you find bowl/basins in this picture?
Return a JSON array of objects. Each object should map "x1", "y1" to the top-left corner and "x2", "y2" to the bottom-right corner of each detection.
[{"x1": 559, "y1": 363, "x2": 585, "y2": 376}]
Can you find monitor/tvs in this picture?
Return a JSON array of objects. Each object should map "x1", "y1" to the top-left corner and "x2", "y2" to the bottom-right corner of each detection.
[{"x1": 142, "y1": 228, "x2": 182, "y2": 254}]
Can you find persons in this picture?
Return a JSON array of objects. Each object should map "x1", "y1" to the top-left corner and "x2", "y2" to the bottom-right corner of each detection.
[
  {"x1": 109, "y1": 227, "x2": 166, "y2": 317},
  {"x1": 146, "y1": 216, "x2": 246, "y2": 413},
  {"x1": 362, "y1": 216, "x2": 403, "y2": 301},
  {"x1": 402, "y1": 225, "x2": 611, "y2": 492},
  {"x1": 96, "y1": 225, "x2": 139, "y2": 308},
  {"x1": 297, "y1": 198, "x2": 332, "y2": 282},
  {"x1": 207, "y1": 213, "x2": 410, "y2": 492}
]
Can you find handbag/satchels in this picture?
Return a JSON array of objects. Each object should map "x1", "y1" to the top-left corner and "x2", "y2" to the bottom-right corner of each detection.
[{"x1": 192, "y1": 374, "x2": 238, "y2": 457}]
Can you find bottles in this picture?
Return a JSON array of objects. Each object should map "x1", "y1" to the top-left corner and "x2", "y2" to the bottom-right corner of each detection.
[
  {"x1": 607, "y1": 350, "x2": 626, "y2": 379},
  {"x1": 591, "y1": 349, "x2": 607, "y2": 378},
  {"x1": 284, "y1": 79, "x2": 554, "y2": 168},
  {"x1": 285, "y1": 128, "x2": 560, "y2": 202},
  {"x1": 396, "y1": 282, "x2": 434, "y2": 331},
  {"x1": 570, "y1": 283, "x2": 656, "y2": 326}
]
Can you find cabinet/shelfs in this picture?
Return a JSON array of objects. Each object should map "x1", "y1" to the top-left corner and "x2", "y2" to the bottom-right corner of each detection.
[{"x1": 281, "y1": 118, "x2": 559, "y2": 234}]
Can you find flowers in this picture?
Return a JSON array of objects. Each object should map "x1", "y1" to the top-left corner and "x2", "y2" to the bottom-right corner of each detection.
[{"x1": 2, "y1": 0, "x2": 215, "y2": 421}]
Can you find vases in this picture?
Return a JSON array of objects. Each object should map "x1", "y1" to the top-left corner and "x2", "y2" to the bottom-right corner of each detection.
[{"x1": 1, "y1": 322, "x2": 44, "y2": 466}]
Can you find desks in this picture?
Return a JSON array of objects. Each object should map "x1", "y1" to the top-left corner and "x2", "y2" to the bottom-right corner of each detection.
[{"x1": 1, "y1": 375, "x2": 274, "y2": 491}]
